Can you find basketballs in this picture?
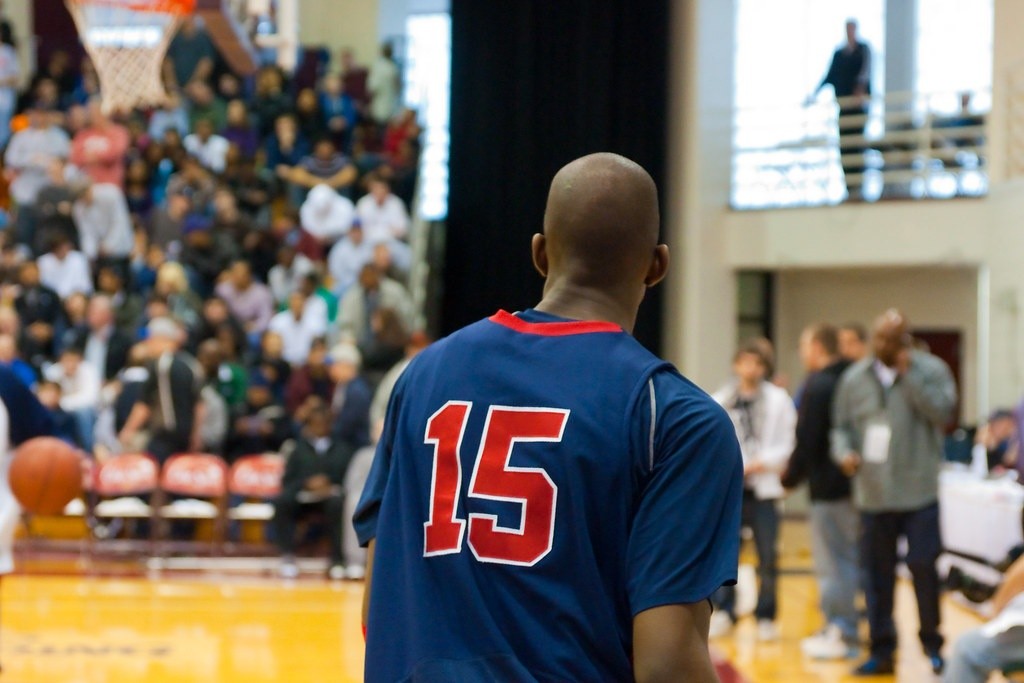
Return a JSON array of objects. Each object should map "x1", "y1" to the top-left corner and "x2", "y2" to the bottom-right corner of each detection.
[{"x1": 8, "y1": 436, "x2": 82, "y2": 514}]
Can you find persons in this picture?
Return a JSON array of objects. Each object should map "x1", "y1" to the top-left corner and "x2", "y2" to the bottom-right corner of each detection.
[
  {"x1": 934, "y1": 553, "x2": 1024, "y2": 683},
  {"x1": 777, "y1": 320, "x2": 864, "y2": 661},
  {"x1": 835, "y1": 323, "x2": 868, "y2": 362},
  {"x1": 0, "y1": 2, "x2": 430, "y2": 582},
  {"x1": 828, "y1": 306, "x2": 962, "y2": 677},
  {"x1": 801, "y1": 16, "x2": 872, "y2": 201},
  {"x1": 968, "y1": 401, "x2": 1024, "y2": 481},
  {"x1": 352, "y1": 151, "x2": 746, "y2": 683},
  {"x1": 707, "y1": 334, "x2": 796, "y2": 642}
]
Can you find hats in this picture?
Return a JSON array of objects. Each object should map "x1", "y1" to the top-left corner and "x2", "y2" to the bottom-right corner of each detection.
[{"x1": 299, "y1": 184, "x2": 356, "y2": 236}]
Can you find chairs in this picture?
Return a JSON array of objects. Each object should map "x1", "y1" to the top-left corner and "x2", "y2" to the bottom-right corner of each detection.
[{"x1": 93, "y1": 447, "x2": 287, "y2": 522}]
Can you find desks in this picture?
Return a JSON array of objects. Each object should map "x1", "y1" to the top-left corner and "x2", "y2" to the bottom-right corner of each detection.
[{"x1": 925, "y1": 457, "x2": 1023, "y2": 568}]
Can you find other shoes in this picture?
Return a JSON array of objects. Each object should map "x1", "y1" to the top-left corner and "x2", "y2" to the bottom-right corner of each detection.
[
  {"x1": 329, "y1": 565, "x2": 345, "y2": 579},
  {"x1": 801, "y1": 623, "x2": 849, "y2": 660},
  {"x1": 347, "y1": 566, "x2": 365, "y2": 578},
  {"x1": 858, "y1": 656, "x2": 893, "y2": 675},
  {"x1": 757, "y1": 619, "x2": 776, "y2": 641},
  {"x1": 282, "y1": 563, "x2": 298, "y2": 577},
  {"x1": 931, "y1": 655, "x2": 944, "y2": 673},
  {"x1": 709, "y1": 610, "x2": 733, "y2": 638}
]
